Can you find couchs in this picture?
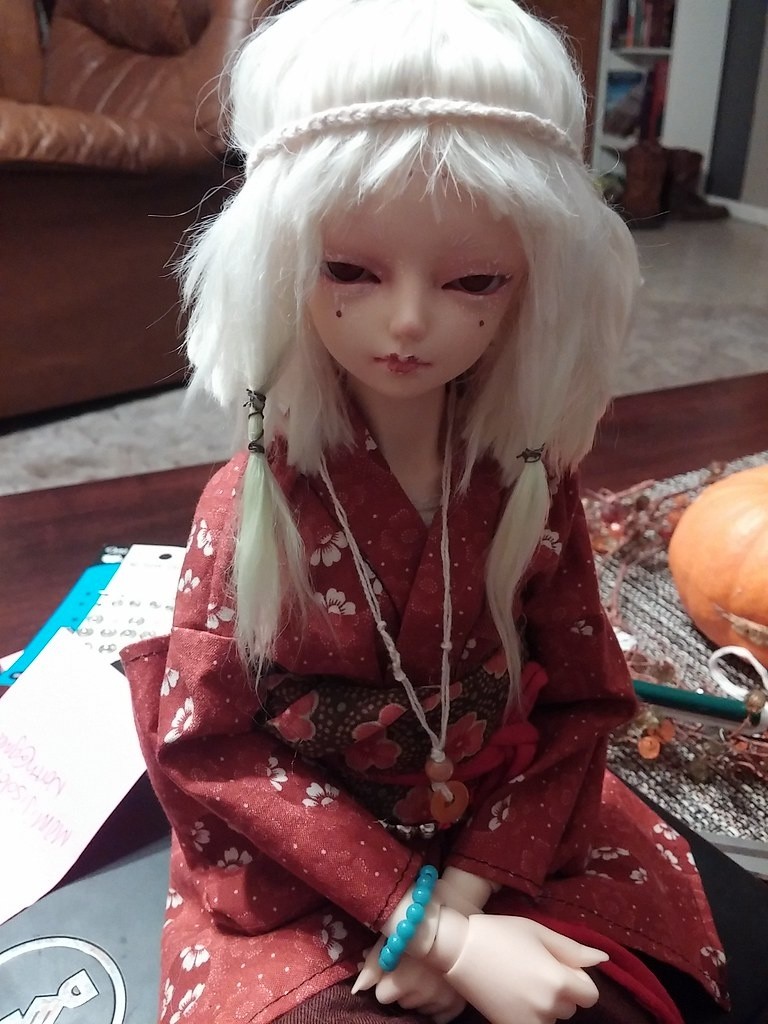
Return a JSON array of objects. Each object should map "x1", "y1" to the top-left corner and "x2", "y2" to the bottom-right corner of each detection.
[{"x1": 0, "y1": 0, "x2": 297, "y2": 444}]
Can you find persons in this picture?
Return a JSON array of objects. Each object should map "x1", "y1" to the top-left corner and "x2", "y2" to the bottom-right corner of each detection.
[{"x1": 119, "y1": 0, "x2": 727, "y2": 1023}]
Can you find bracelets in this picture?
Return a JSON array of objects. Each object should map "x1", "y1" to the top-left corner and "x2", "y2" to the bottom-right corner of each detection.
[{"x1": 379, "y1": 865, "x2": 439, "y2": 971}]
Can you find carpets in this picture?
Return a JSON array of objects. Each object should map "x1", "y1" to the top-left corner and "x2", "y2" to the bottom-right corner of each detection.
[{"x1": 583, "y1": 445, "x2": 768, "y2": 879}]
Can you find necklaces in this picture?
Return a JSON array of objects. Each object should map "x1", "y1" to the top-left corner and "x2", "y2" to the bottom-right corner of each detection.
[{"x1": 304, "y1": 380, "x2": 469, "y2": 826}]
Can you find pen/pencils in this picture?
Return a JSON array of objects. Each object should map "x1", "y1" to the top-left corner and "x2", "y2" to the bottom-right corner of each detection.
[{"x1": 633, "y1": 680, "x2": 768, "y2": 733}]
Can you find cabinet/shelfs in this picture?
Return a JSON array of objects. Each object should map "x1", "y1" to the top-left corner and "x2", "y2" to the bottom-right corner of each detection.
[{"x1": 531, "y1": 0, "x2": 678, "y2": 193}]
